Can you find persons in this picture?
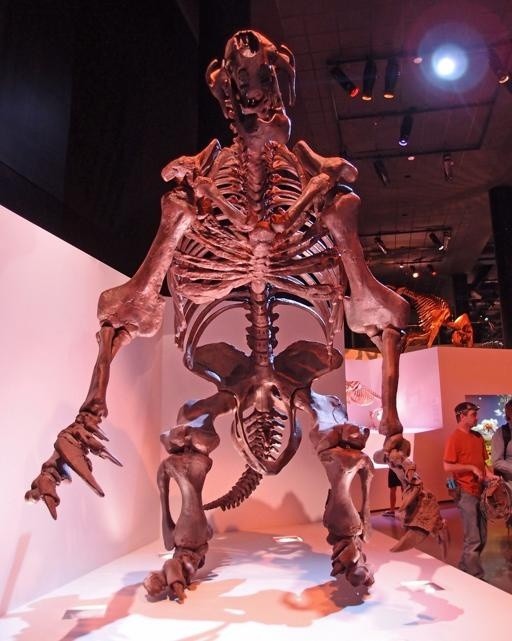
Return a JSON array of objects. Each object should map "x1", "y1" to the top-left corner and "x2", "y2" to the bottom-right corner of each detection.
[
  {"x1": 442, "y1": 402, "x2": 503, "y2": 583},
  {"x1": 491, "y1": 399, "x2": 512, "y2": 529},
  {"x1": 381, "y1": 451, "x2": 410, "y2": 516}
]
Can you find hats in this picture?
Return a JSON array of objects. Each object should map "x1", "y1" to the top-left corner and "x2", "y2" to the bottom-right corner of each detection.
[{"x1": 455, "y1": 402, "x2": 479, "y2": 414}]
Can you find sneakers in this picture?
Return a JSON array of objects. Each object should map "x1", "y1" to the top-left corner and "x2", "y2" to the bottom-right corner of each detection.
[{"x1": 382, "y1": 510, "x2": 396, "y2": 517}]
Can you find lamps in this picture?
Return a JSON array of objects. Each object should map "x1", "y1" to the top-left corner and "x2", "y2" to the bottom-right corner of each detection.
[
  {"x1": 360, "y1": 58, "x2": 376, "y2": 101},
  {"x1": 326, "y1": 57, "x2": 358, "y2": 97},
  {"x1": 397, "y1": 113, "x2": 412, "y2": 147},
  {"x1": 374, "y1": 239, "x2": 389, "y2": 256},
  {"x1": 485, "y1": 50, "x2": 510, "y2": 86},
  {"x1": 410, "y1": 264, "x2": 420, "y2": 280},
  {"x1": 428, "y1": 232, "x2": 445, "y2": 252},
  {"x1": 374, "y1": 161, "x2": 390, "y2": 187},
  {"x1": 381, "y1": 56, "x2": 399, "y2": 100},
  {"x1": 426, "y1": 262, "x2": 437, "y2": 276},
  {"x1": 442, "y1": 154, "x2": 454, "y2": 182}
]
